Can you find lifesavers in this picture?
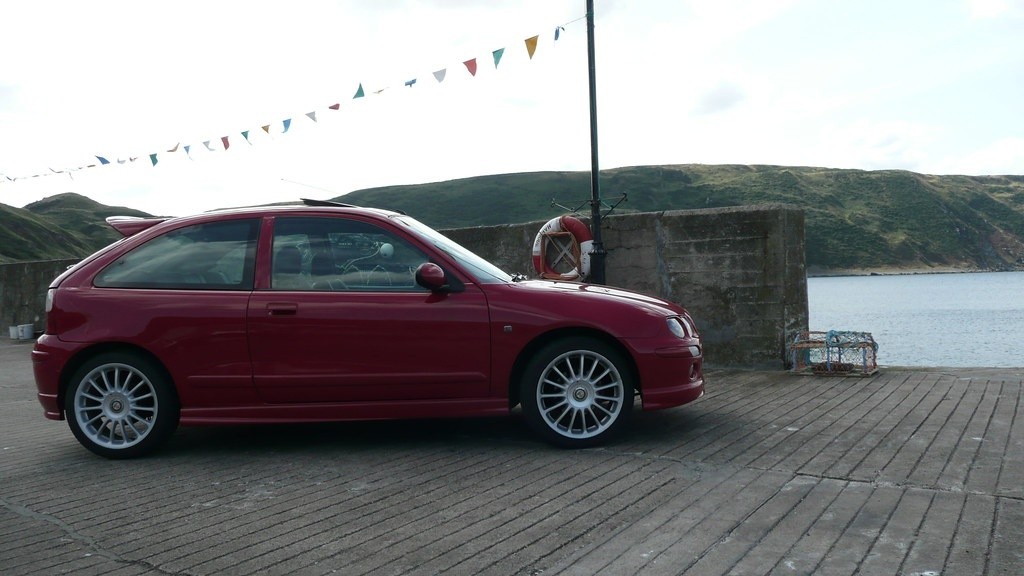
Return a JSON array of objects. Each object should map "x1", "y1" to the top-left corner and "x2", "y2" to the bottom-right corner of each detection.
[{"x1": 531, "y1": 214, "x2": 595, "y2": 283}]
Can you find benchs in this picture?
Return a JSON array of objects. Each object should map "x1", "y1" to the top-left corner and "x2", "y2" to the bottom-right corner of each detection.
[{"x1": 153, "y1": 270, "x2": 235, "y2": 284}]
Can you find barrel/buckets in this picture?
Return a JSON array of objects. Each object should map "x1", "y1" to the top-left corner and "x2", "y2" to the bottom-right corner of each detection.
[
  {"x1": 9, "y1": 326, "x2": 19, "y2": 339},
  {"x1": 17, "y1": 324, "x2": 34, "y2": 339}
]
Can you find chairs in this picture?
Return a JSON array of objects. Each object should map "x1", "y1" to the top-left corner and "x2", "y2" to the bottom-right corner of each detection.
[
  {"x1": 273, "y1": 246, "x2": 312, "y2": 290},
  {"x1": 311, "y1": 251, "x2": 336, "y2": 275}
]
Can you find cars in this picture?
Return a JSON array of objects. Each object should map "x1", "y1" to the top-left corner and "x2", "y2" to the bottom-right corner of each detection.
[
  {"x1": 276, "y1": 230, "x2": 417, "y2": 279},
  {"x1": 30, "y1": 197, "x2": 705, "y2": 460}
]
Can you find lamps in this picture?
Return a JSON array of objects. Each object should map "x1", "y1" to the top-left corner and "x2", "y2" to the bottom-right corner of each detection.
[{"x1": 345, "y1": 240, "x2": 395, "y2": 272}]
